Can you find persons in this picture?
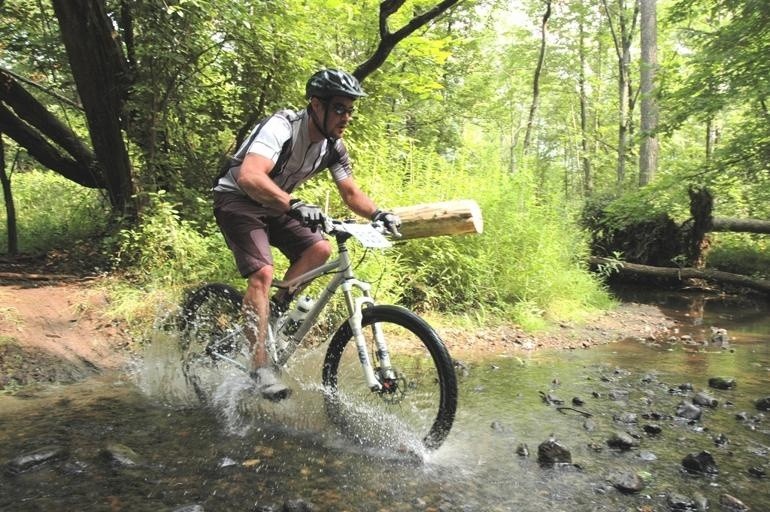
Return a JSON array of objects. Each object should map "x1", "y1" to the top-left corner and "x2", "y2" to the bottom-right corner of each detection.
[{"x1": 212, "y1": 68, "x2": 405, "y2": 402}]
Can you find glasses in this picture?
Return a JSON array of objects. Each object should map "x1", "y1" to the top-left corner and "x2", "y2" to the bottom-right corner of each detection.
[{"x1": 331, "y1": 103, "x2": 355, "y2": 116}]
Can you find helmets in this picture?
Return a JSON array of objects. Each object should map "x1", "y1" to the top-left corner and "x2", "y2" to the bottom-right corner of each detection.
[{"x1": 305, "y1": 69, "x2": 368, "y2": 102}]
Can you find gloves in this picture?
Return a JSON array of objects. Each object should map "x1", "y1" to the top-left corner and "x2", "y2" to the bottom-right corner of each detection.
[
  {"x1": 371, "y1": 209, "x2": 403, "y2": 239},
  {"x1": 286, "y1": 198, "x2": 333, "y2": 234}
]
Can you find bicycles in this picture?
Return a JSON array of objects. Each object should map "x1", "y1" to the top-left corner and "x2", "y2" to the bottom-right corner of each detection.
[{"x1": 178, "y1": 209, "x2": 457, "y2": 466}]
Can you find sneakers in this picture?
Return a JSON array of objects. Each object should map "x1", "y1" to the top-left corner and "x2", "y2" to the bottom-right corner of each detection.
[
  {"x1": 248, "y1": 368, "x2": 292, "y2": 401},
  {"x1": 284, "y1": 291, "x2": 338, "y2": 324}
]
